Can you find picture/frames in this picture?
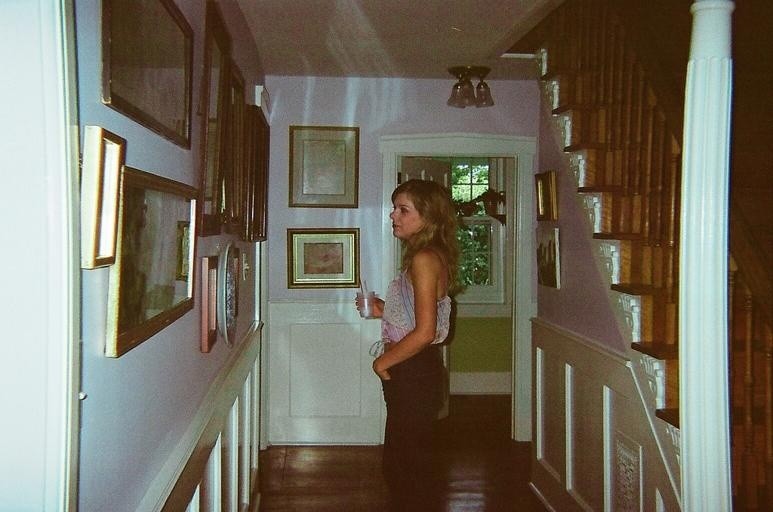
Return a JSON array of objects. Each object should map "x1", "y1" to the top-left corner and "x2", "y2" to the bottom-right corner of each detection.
[
  {"x1": 95, "y1": 0, "x2": 197, "y2": 152},
  {"x1": 284, "y1": 226, "x2": 362, "y2": 291},
  {"x1": 286, "y1": 125, "x2": 360, "y2": 209},
  {"x1": 103, "y1": 163, "x2": 202, "y2": 363},
  {"x1": 533, "y1": 170, "x2": 559, "y2": 221},
  {"x1": 77, "y1": 125, "x2": 127, "y2": 268},
  {"x1": 195, "y1": 0, "x2": 272, "y2": 357}
]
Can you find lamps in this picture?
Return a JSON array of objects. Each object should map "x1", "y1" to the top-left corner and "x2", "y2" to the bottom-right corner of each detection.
[{"x1": 446, "y1": 65, "x2": 496, "y2": 110}]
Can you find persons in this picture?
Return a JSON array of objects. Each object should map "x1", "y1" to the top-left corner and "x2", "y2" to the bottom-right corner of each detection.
[{"x1": 354, "y1": 178, "x2": 464, "y2": 511}]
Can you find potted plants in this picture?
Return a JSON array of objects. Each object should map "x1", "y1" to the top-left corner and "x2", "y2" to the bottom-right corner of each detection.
[{"x1": 452, "y1": 187, "x2": 503, "y2": 217}]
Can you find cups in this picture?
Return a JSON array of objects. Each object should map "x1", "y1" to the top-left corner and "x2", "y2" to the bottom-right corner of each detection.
[{"x1": 355, "y1": 291, "x2": 375, "y2": 319}]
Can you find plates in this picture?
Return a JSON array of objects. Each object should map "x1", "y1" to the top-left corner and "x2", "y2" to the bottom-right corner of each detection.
[{"x1": 216, "y1": 240, "x2": 240, "y2": 348}]
